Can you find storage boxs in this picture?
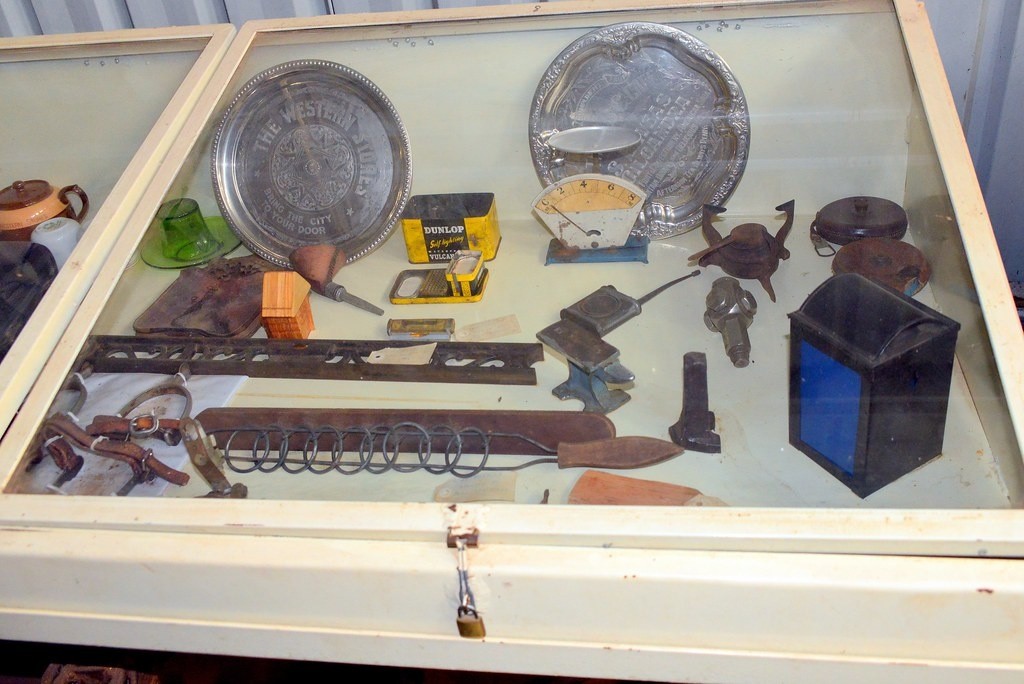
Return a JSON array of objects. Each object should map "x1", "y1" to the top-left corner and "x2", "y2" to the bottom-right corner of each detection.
[{"x1": 399, "y1": 192, "x2": 501, "y2": 267}]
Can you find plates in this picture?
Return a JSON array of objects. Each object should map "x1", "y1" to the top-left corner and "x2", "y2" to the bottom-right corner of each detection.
[{"x1": 141, "y1": 215, "x2": 243, "y2": 268}]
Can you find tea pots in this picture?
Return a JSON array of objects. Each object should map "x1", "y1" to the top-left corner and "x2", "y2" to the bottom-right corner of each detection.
[{"x1": 0, "y1": 179, "x2": 90, "y2": 241}]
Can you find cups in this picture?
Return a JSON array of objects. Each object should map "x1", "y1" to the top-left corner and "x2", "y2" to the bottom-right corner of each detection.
[
  {"x1": 155, "y1": 198, "x2": 217, "y2": 259},
  {"x1": 30, "y1": 216, "x2": 85, "y2": 273}
]
